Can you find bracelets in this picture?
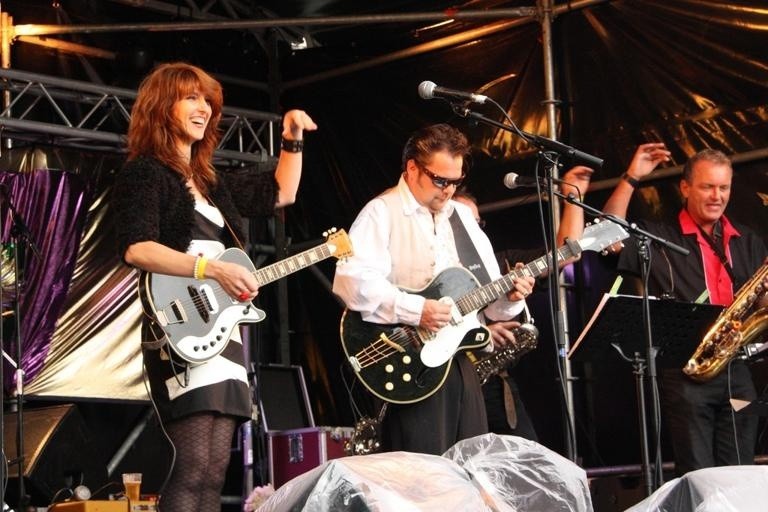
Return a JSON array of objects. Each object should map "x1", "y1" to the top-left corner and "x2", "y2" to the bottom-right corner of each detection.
[
  {"x1": 280, "y1": 136, "x2": 304, "y2": 154},
  {"x1": 620, "y1": 169, "x2": 640, "y2": 189},
  {"x1": 193, "y1": 256, "x2": 200, "y2": 279},
  {"x1": 197, "y1": 256, "x2": 208, "y2": 279}
]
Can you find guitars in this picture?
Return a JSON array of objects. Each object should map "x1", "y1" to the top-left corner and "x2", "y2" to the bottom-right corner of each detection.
[
  {"x1": 137, "y1": 228, "x2": 357, "y2": 367},
  {"x1": 340, "y1": 218, "x2": 633, "y2": 405}
]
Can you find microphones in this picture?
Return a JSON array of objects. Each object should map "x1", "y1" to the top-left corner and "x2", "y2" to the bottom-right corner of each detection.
[
  {"x1": 503, "y1": 173, "x2": 563, "y2": 190},
  {"x1": 417, "y1": 80, "x2": 486, "y2": 104}
]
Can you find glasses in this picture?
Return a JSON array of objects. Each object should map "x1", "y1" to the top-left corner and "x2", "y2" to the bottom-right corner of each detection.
[{"x1": 415, "y1": 159, "x2": 466, "y2": 192}]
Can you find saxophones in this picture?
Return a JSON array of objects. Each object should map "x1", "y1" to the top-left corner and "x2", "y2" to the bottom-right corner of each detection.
[{"x1": 682, "y1": 264, "x2": 768, "y2": 381}]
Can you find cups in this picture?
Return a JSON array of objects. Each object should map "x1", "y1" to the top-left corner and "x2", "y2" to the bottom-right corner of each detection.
[{"x1": 122, "y1": 476, "x2": 142, "y2": 501}]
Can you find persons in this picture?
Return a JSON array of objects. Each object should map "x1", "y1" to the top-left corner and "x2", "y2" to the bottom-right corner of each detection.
[
  {"x1": 600, "y1": 141, "x2": 768, "y2": 472},
  {"x1": 556, "y1": 167, "x2": 611, "y2": 296},
  {"x1": 325, "y1": 122, "x2": 537, "y2": 454},
  {"x1": 112, "y1": 60, "x2": 317, "y2": 512},
  {"x1": 453, "y1": 165, "x2": 593, "y2": 446}
]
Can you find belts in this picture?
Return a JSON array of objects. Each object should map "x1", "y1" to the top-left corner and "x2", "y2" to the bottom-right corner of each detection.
[{"x1": 498, "y1": 369, "x2": 518, "y2": 430}]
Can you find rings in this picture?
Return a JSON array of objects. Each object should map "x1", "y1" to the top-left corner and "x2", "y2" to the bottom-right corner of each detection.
[
  {"x1": 237, "y1": 292, "x2": 249, "y2": 302},
  {"x1": 520, "y1": 289, "x2": 529, "y2": 298}
]
